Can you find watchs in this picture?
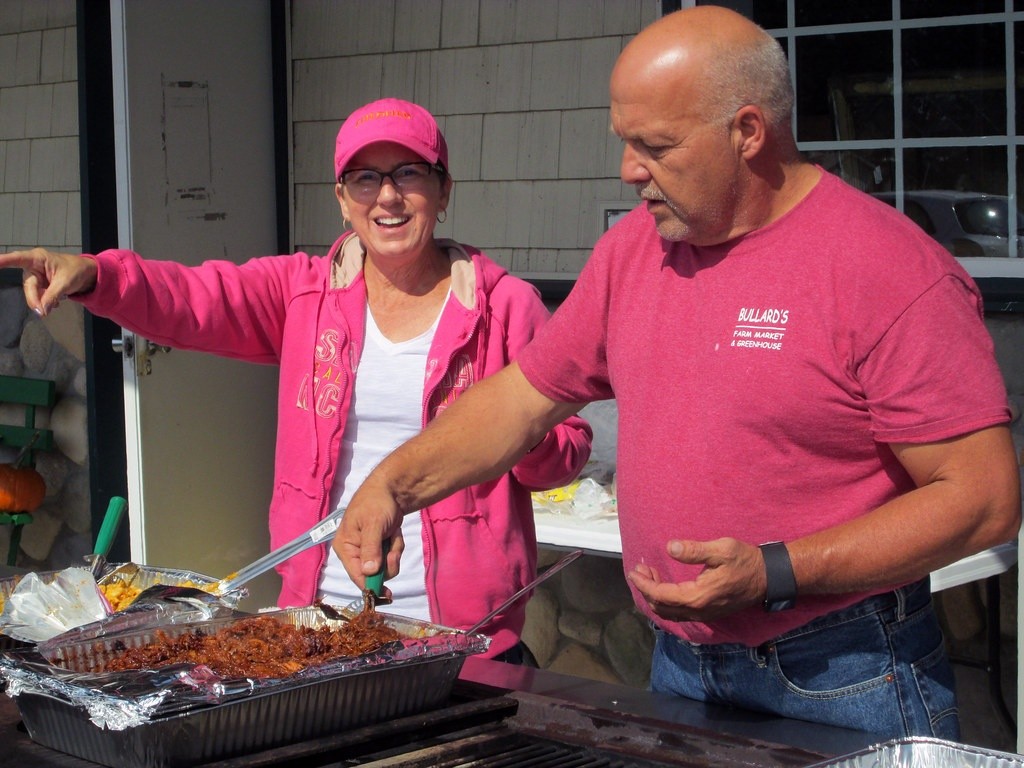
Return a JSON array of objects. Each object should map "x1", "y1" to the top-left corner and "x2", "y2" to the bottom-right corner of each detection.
[{"x1": 757, "y1": 541, "x2": 797, "y2": 613}]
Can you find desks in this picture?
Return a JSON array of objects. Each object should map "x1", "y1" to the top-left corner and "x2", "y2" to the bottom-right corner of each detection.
[{"x1": 529, "y1": 462, "x2": 1018, "y2": 754}]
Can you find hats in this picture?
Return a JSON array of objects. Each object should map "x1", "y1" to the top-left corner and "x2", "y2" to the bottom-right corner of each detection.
[{"x1": 334, "y1": 98, "x2": 448, "y2": 181}]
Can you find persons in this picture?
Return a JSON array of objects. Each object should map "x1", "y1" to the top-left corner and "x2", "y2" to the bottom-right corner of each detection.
[
  {"x1": 331, "y1": 6, "x2": 1022, "y2": 741},
  {"x1": 1, "y1": 99, "x2": 594, "y2": 665}
]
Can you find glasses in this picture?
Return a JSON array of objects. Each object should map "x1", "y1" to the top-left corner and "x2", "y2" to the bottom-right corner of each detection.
[{"x1": 339, "y1": 163, "x2": 444, "y2": 192}]
